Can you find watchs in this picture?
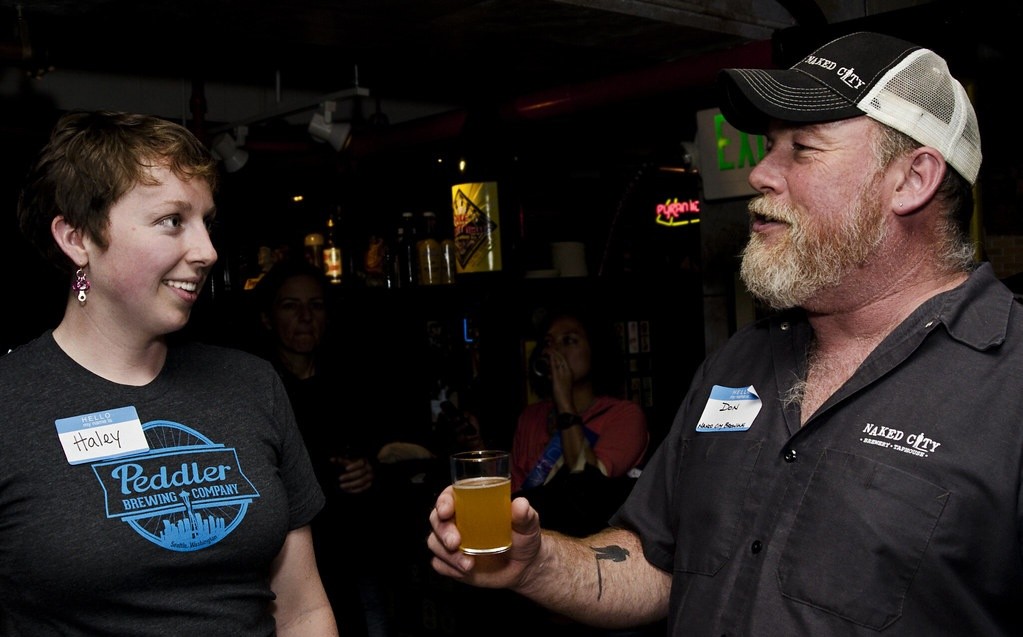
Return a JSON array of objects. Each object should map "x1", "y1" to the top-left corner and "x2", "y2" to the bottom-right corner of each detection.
[{"x1": 555, "y1": 413, "x2": 583, "y2": 427}]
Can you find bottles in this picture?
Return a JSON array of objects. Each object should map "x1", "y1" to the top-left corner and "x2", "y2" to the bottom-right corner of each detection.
[
  {"x1": 400, "y1": 212, "x2": 418, "y2": 285},
  {"x1": 395, "y1": 229, "x2": 404, "y2": 285},
  {"x1": 381, "y1": 252, "x2": 394, "y2": 289},
  {"x1": 441, "y1": 233, "x2": 457, "y2": 285},
  {"x1": 320, "y1": 216, "x2": 343, "y2": 286},
  {"x1": 416, "y1": 212, "x2": 443, "y2": 284}
]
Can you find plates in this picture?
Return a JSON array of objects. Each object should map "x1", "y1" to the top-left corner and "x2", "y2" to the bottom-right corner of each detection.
[{"x1": 552, "y1": 242, "x2": 586, "y2": 277}]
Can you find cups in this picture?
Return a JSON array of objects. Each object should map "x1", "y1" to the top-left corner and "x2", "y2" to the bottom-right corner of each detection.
[
  {"x1": 450, "y1": 450, "x2": 512, "y2": 556},
  {"x1": 534, "y1": 354, "x2": 551, "y2": 376}
]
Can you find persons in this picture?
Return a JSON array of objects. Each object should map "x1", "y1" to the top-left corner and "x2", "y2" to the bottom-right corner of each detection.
[
  {"x1": 257, "y1": 276, "x2": 372, "y2": 494},
  {"x1": 427, "y1": 31, "x2": 1023, "y2": 637},
  {"x1": 505, "y1": 314, "x2": 650, "y2": 497},
  {"x1": 0, "y1": 108, "x2": 343, "y2": 637}
]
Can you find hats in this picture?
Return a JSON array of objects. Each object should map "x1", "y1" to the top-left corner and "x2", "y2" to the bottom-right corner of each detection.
[{"x1": 713, "y1": 31, "x2": 985, "y2": 189}]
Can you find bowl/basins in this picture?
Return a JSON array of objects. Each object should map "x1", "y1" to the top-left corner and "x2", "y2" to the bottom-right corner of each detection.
[{"x1": 527, "y1": 269, "x2": 559, "y2": 277}]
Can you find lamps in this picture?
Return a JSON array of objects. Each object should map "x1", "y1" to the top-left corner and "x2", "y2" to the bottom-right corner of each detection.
[
  {"x1": 306, "y1": 101, "x2": 358, "y2": 152},
  {"x1": 207, "y1": 128, "x2": 250, "y2": 171}
]
